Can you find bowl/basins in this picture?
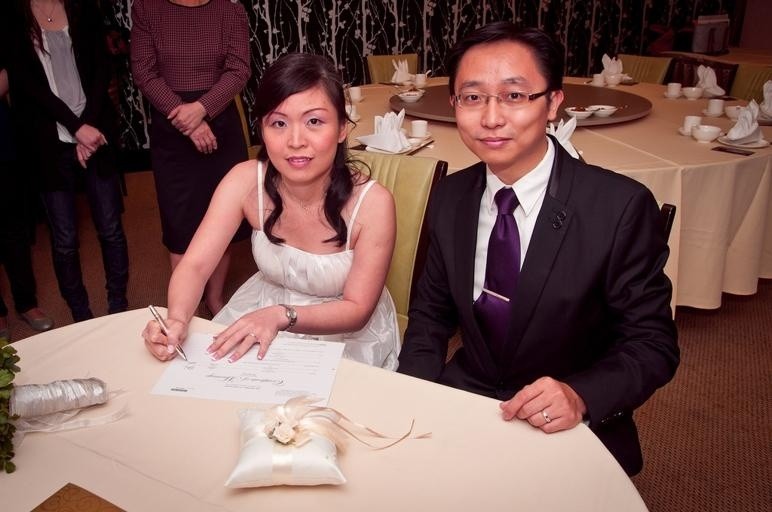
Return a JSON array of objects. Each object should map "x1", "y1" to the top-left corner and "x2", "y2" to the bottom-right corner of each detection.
[
  {"x1": 725, "y1": 105, "x2": 745, "y2": 121},
  {"x1": 409, "y1": 139, "x2": 422, "y2": 146},
  {"x1": 566, "y1": 107, "x2": 592, "y2": 119},
  {"x1": 683, "y1": 88, "x2": 700, "y2": 100},
  {"x1": 589, "y1": 104, "x2": 617, "y2": 118},
  {"x1": 691, "y1": 125, "x2": 720, "y2": 143},
  {"x1": 399, "y1": 93, "x2": 423, "y2": 103}
]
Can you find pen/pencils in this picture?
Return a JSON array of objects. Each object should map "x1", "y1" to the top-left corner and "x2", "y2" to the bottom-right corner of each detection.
[{"x1": 147, "y1": 304, "x2": 188, "y2": 361}]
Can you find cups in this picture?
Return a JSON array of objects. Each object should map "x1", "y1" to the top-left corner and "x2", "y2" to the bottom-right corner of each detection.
[
  {"x1": 348, "y1": 86, "x2": 361, "y2": 102},
  {"x1": 668, "y1": 83, "x2": 681, "y2": 97},
  {"x1": 684, "y1": 115, "x2": 701, "y2": 131},
  {"x1": 415, "y1": 74, "x2": 425, "y2": 85},
  {"x1": 708, "y1": 99, "x2": 722, "y2": 113},
  {"x1": 346, "y1": 105, "x2": 357, "y2": 116},
  {"x1": 412, "y1": 121, "x2": 427, "y2": 138}
]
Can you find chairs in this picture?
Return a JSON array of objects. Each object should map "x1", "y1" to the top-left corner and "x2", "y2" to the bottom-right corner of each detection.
[
  {"x1": 346, "y1": 148, "x2": 449, "y2": 352},
  {"x1": 661, "y1": 45, "x2": 772, "y2": 103},
  {"x1": 614, "y1": 54, "x2": 676, "y2": 86},
  {"x1": 363, "y1": 53, "x2": 422, "y2": 84}
]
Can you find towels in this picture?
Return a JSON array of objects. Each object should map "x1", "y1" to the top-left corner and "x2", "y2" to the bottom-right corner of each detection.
[
  {"x1": 390, "y1": 57, "x2": 415, "y2": 84},
  {"x1": 601, "y1": 52, "x2": 623, "y2": 77},
  {"x1": 355, "y1": 107, "x2": 412, "y2": 153},
  {"x1": 760, "y1": 79, "x2": 772, "y2": 119},
  {"x1": 696, "y1": 63, "x2": 726, "y2": 98},
  {"x1": 725, "y1": 97, "x2": 764, "y2": 146},
  {"x1": 547, "y1": 115, "x2": 581, "y2": 160}
]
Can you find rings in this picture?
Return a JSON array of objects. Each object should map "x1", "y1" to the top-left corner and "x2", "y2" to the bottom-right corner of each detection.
[
  {"x1": 249, "y1": 334, "x2": 258, "y2": 340},
  {"x1": 540, "y1": 409, "x2": 553, "y2": 424}
]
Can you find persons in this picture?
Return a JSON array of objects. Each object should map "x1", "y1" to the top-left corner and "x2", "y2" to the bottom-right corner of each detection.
[
  {"x1": 21, "y1": 0, "x2": 129, "y2": 324},
  {"x1": 0, "y1": 69, "x2": 53, "y2": 341},
  {"x1": 130, "y1": 0, "x2": 251, "y2": 320},
  {"x1": 142, "y1": 52, "x2": 400, "y2": 375},
  {"x1": 396, "y1": 24, "x2": 682, "y2": 476}
]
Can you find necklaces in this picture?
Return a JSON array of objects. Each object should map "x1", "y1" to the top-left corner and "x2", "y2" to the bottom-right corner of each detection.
[{"x1": 32, "y1": 2, "x2": 57, "y2": 22}]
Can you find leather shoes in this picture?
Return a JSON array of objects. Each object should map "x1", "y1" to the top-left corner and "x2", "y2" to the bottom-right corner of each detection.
[
  {"x1": 17, "y1": 312, "x2": 55, "y2": 332},
  {"x1": 0, "y1": 315, "x2": 12, "y2": 343}
]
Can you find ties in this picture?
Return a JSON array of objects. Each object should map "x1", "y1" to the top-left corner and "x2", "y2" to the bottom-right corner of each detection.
[{"x1": 473, "y1": 187, "x2": 522, "y2": 355}]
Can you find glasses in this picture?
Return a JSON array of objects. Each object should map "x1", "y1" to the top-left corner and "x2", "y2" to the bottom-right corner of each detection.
[{"x1": 453, "y1": 90, "x2": 548, "y2": 108}]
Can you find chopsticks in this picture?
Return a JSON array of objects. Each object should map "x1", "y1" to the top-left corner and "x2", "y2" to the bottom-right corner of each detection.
[{"x1": 408, "y1": 140, "x2": 437, "y2": 155}]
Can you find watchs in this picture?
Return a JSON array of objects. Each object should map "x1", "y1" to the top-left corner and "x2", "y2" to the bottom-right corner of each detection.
[{"x1": 278, "y1": 303, "x2": 298, "y2": 332}]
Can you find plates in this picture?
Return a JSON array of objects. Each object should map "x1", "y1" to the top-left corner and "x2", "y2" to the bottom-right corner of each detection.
[
  {"x1": 679, "y1": 128, "x2": 690, "y2": 136},
  {"x1": 408, "y1": 131, "x2": 432, "y2": 140},
  {"x1": 703, "y1": 109, "x2": 723, "y2": 118},
  {"x1": 717, "y1": 135, "x2": 768, "y2": 148},
  {"x1": 366, "y1": 146, "x2": 411, "y2": 154}
]
